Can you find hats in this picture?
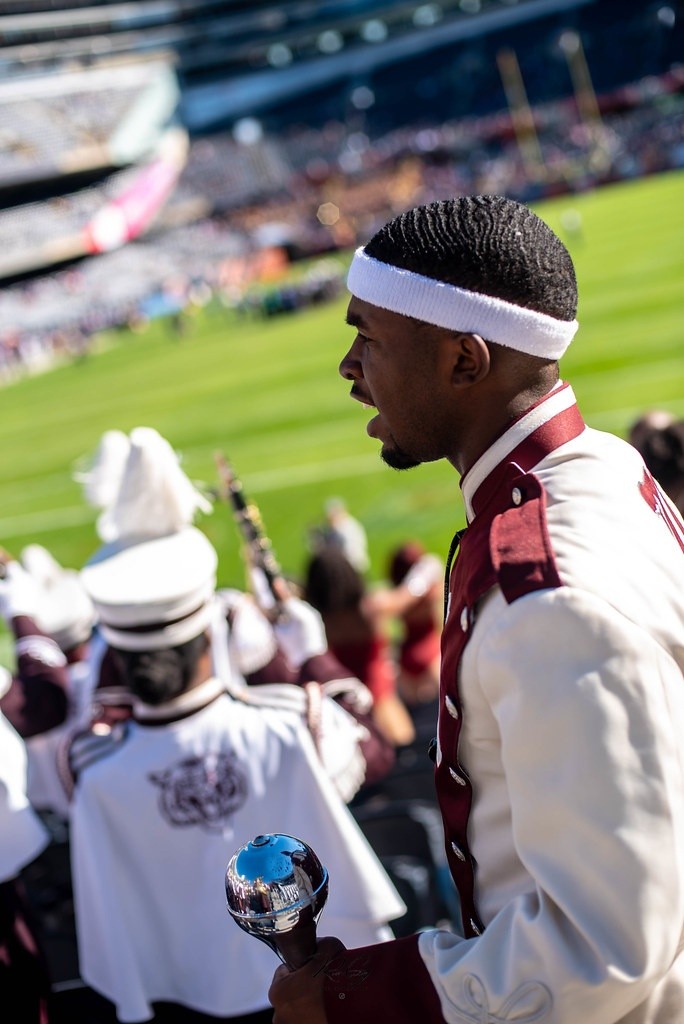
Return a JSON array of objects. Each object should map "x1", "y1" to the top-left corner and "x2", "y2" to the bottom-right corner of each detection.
[
  {"x1": 218, "y1": 588, "x2": 276, "y2": 673},
  {"x1": 20, "y1": 545, "x2": 99, "y2": 647},
  {"x1": 74, "y1": 426, "x2": 218, "y2": 652}
]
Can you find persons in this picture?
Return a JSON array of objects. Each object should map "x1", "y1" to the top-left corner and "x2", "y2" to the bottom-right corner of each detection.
[
  {"x1": 269, "y1": 194, "x2": 684, "y2": 1024},
  {"x1": 0, "y1": 49, "x2": 684, "y2": 1024}
]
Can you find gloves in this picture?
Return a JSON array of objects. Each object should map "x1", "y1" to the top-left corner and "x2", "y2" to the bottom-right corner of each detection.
[
  {"x1": 276, "y1": 600, "x2": 329, "y2": 667},
  {"x1": 1, "y1": 560, "x2": 33, "y2": 619}
]
